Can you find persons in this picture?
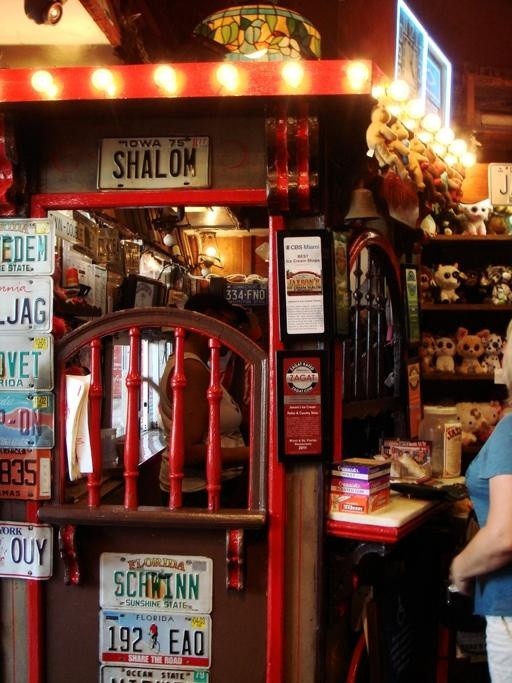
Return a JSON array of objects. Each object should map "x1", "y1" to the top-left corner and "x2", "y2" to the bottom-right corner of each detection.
[
  {"x1": 447, "y1": 315, "x2": 512, "y2": 681},
  {"x1": 157, "y1": 288, "x2": 248, "y2": 510}
]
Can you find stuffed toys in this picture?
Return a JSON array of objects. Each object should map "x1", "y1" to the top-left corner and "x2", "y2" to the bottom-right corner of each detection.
[{"x1": 414, "y1": 198, "x2": 512, "y2": 448}]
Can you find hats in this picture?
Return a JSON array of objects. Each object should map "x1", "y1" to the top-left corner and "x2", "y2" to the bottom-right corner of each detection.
[{"x1": 183, "y1": 293, "x2": 248, "y2": 324}]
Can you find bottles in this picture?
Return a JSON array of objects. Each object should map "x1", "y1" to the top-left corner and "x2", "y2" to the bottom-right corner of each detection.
[
  {"x1": 416, "y1": 404, "x2": 463, "y2": 481},
  {"x1": 63, "y1": 267, "x2": 89, "y2": 321}
]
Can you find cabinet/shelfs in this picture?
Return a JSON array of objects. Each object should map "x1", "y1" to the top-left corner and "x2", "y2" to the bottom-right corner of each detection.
[{"x1": 412, "y1": 218, "x2": 511, "y2": 454}]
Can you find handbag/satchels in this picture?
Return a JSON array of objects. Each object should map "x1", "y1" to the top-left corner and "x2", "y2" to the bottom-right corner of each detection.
[
  {"x1": 389, "y1": 483, "x2": 436, "y2": 500},
  {"x1": 445, "y1": 581, "x2": 486, "y2": 631}
]
